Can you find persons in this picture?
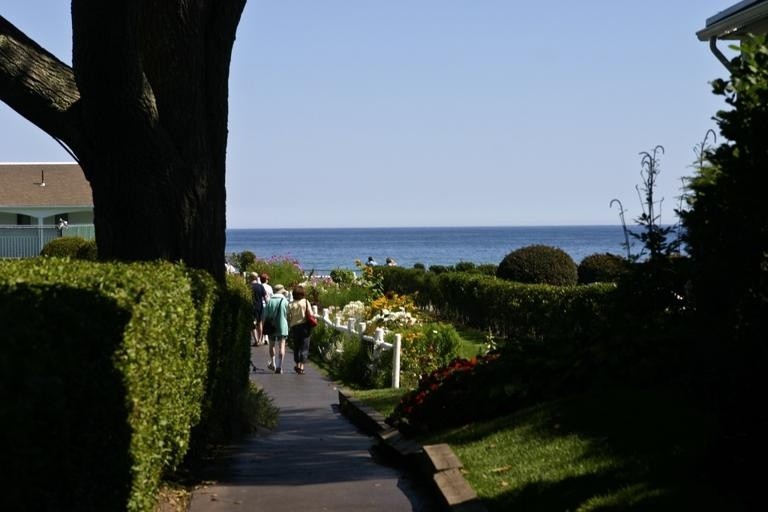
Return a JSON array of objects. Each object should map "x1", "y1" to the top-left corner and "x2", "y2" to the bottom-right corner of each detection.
[
  {"x1": 56, "y1": 217, "x2": 68, "y2": 230},
  {"x1": 383, "y1": 257, "x2": 397, "y2": 266},
  {"x1": 364, "y1": 256, "x2": 377, "y2": 266},
  {"x1": 224, "y1": 258, "x2": 314, "y2": 375}
]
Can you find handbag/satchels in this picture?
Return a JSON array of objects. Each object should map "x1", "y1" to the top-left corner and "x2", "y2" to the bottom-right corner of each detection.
[
  {"x1": 304, "y1": 299, "x2": 319, "y2": 327},
  {"x1": 262, "y1": 317, "x2": 276, "y2": 335}
]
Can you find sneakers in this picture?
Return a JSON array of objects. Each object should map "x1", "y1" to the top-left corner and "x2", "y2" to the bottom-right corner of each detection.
[
  {"x1": 293, "y1": 365, "x2": 305, "y2": 373},
  {"x1": 252, "y1": 339, "x2": 269, "y2": 347},
  {"x1": 268, "y1": 360, "x2": 276, "y2": 370},
  {"x1": 276, "y1": 367, "x2": 283, "y2": 374}
]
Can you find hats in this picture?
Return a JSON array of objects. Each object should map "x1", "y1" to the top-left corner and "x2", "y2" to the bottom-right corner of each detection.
[{"x1": 273, "y1": 285, "x2": 286, "y2": 294}]
